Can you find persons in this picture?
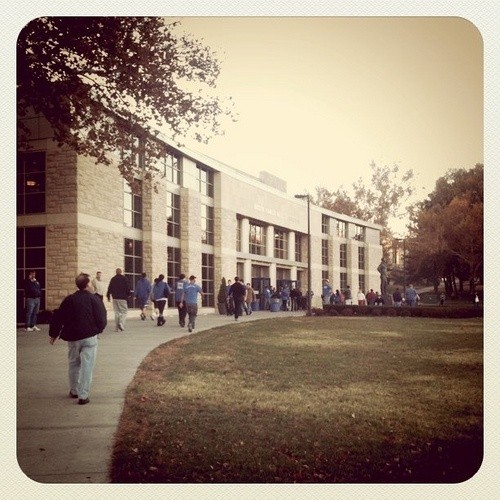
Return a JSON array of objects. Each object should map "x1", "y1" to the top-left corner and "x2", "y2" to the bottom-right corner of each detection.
[
  {"x1": 357, "y1": 288, "x2": 376, "y2": 306},
  {"x1": 228, "y1": 276, "x2": 245, "y2": 320},
  {"x1": 150, "y1": 274, "x2": 173, "y2": 326},
  {"x1": 393, "y1": 287, "x2": 402, "y2": 306},
  {"x1": 377, "y1": 257, "x2": 387, "y2": 295},
  {"x1": 179, "y1": 275, "x2": 204, "y2": 332},
  {"x1": 473, "y1": 292, "x2": 480, "y2": 306},
  {"x1": 91, "y1": 271, "x2": 104, "y2": 300},
  {"x1": 440, "y1": 291, "x2": 446, "y2": 305},
  {"x1": 281, "y1": 288, "x2": 289, "y2": 311},
  {"x1": 262, "y1": 286, "x2": 271, "y2": 310},
  {"x1": 403, "y1": 284, "x2": 417, "y2": 307},
  {"x1": 49, "y1": 272, "x2": 108, "y2": 404},
  {"x1": 132, "y1": 272, "x2": 151, "y2": 319},
  {"x1": 225, "y1": 279, "x2": 255, "y2": 316},
  {"x1": 106, "y1": 267, "x2": 130, "y2": 332},
  {"x1": 321, "y1": 285, "x2": 352, "y2": 304},
  {"x1": 24, "y1": 269, "x2": 42, "y2": 331},
  {"x1": 176, "y1": 274, "x2": 189, "y2": 327},
  {"x1": 323, "y1": 279, "x2": 333, "y2": 304}
]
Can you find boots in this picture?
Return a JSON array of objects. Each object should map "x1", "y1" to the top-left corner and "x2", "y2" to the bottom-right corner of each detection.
[{"x1": 157, "y1": 316, "x2": 166, "y2": 326}]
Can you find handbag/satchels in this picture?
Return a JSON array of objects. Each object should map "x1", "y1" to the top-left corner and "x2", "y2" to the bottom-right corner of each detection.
[{"x1": 164, "y1": 282, "x2": 170, "y2": 298}]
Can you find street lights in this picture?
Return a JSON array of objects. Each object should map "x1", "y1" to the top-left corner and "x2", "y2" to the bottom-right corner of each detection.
[
  {"x1": 395, "y1": 239, "x2": 406, "y2": 296},
  {"x1": 295, "y1": 194, "x2": 312, "y2": 316}
]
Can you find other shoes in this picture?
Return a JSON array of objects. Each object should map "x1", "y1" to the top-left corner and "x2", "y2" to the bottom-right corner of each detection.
[
  {"x1": 118, "y1": 323, "x2": 124, "y2": 331},
  {"x1": 24, "y1": 327, "x2": 33, "y2": 331},
  {"x1": 70, "y1": 391, "x2": 79, "y2": 398},
  {"x1": 78, "y1": 398, "x2": 90, "y2": 405},
  {"x1": 116, "y1": 329, "x2": 121, "y2": 331},
  {"x1": 188, "y1": 323, "x2": 192, "y2": 332},
  {"x1": 140, "y1": 313, "x2": 146, "y2": 320},
  {"x1": 31, "y1": 325, "x2": 40, "y2": 331}
]
